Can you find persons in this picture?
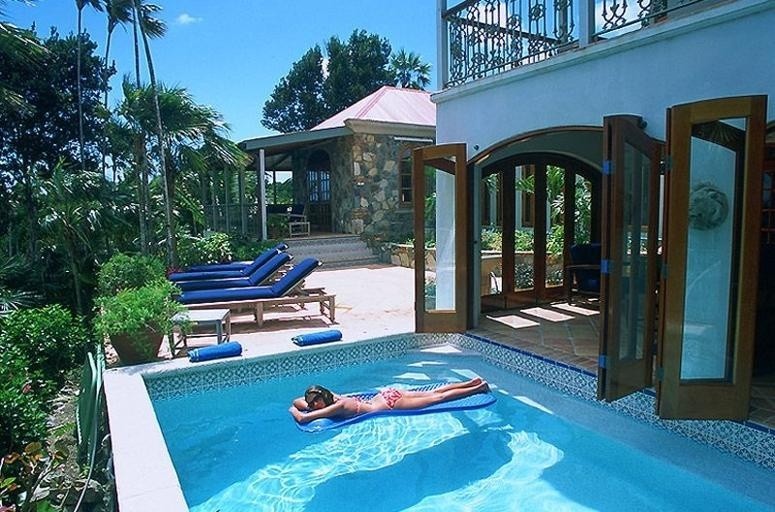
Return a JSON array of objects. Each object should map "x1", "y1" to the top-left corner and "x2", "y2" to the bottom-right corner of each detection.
[{"x1": 288, "y1": 376, "x2": 492, "y2": 423}]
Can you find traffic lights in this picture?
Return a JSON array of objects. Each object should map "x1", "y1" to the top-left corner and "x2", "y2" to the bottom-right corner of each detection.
[{"x1": 294, "y1": 329, "x2": 344, "y2": 347}]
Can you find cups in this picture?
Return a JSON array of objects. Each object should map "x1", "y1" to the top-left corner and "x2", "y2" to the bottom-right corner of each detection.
[{"x1": 307, "y1": 395, "x2": 321, "y2": 409}]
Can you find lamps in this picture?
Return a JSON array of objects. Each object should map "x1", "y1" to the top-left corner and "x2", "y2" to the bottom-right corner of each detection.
[
  {"x1": 569, "y1": 242, "x2": 634, "y2": 328},
  {"x1": 267, "y1": 196, "x2": 311, "y2": 238},
  {"x1": 166, "y1": 243, "x2": 338, "y2": 358}
]
